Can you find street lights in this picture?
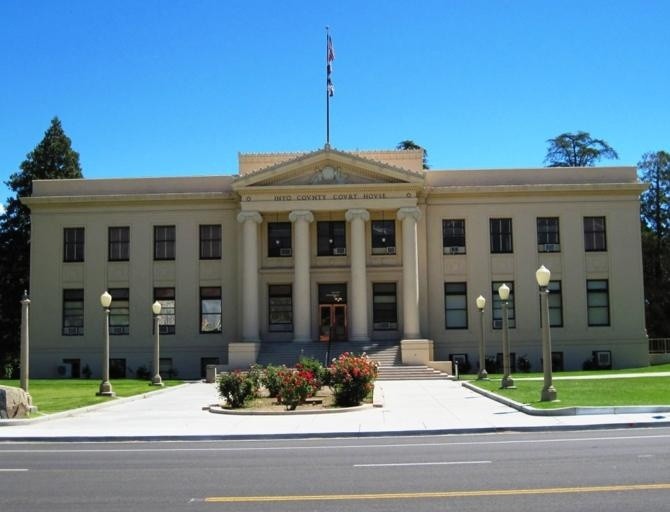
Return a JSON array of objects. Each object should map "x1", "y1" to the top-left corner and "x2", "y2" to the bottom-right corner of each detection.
[
  {"x1": 476, "y1": 295, "x2": 490, "y2": 382},
  {"x1": 498, "y1": 283, "x2": 517, "y2": 389},
  {"x1": 149, "y1": 300, "x2": 164, "y2": 387},
  {"x1": 535, "y1": 264, "x2": 561, "y2": 403},
  {"x1": 19, "y1": 288, "x2": 32, "y2": 393},
  {"x1": 96, "y1": 291, "x2": 116, "y2": 397}
]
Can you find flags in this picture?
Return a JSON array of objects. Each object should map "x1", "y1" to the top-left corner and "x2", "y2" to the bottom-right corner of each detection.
[{"x1": 328, "y1": 35, "x2": 335, "y2": 63}]
